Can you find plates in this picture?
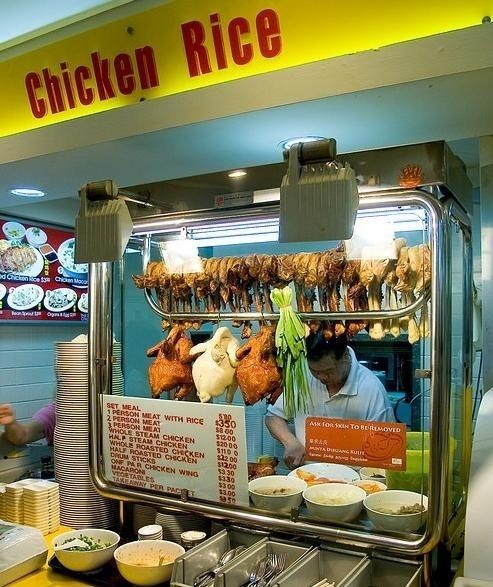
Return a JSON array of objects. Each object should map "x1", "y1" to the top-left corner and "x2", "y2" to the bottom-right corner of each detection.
[
  {"x1": 132, "y1": 502, "x2": 208, "y2": 550},
  {"x1": 0, "y1": 342, "x2": 125, "y2": 536},
  {"x1": 0, "y1": 238, "x2": 88, "y2": 315}
]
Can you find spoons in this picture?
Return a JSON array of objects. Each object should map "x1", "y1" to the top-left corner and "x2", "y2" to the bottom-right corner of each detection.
[{"x1": 194, "y1": 544, "x2": 245, "y2": 587}]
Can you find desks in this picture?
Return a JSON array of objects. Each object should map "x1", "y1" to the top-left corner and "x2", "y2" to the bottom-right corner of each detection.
[{"x1": 1, "y1": 525, "x2": 102, "y2": 587}]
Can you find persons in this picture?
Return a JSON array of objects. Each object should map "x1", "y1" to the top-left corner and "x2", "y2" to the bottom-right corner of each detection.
[
  {"x1": 0, "y1": 401, "x2": 56, "y2": 447},
  {"x1": 264, "y1": 317, "x2": 397, "y2": 471}
]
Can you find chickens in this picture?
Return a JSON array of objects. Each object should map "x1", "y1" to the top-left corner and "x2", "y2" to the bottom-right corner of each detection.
[
  {"x1": 145, "y1": 323, "x2": 196, "y2": 401},
  {"x1": 234, "y1": 324, "x2": 285, "y2": 406},
  {"x1": 188, "y1": 326, "x2": 241, "y2": 404}
]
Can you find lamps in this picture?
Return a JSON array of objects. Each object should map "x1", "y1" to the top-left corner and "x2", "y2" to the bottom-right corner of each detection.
[
  {"x1": 76, "y1": 180, "x2": 134, "y2": 262},
  {"x1": 279, "y1": 138, "x2": 358, "y2": 243}
]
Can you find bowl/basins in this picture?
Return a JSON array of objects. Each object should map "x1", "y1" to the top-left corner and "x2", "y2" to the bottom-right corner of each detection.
[
  {"x1": 249, "y1": 463, "x2": 431, "y2": 533},
  {"x1": 2, "y1": 222, "x2": 47, "y2": 247},
  {"x1": 52, "y1": 528, "x2": 186, "y2": 587}
]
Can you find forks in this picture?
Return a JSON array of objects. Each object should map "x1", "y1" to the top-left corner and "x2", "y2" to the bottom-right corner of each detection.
[{"x1": 244, "y1": 552, "x2": 287, "y2": 587}]
